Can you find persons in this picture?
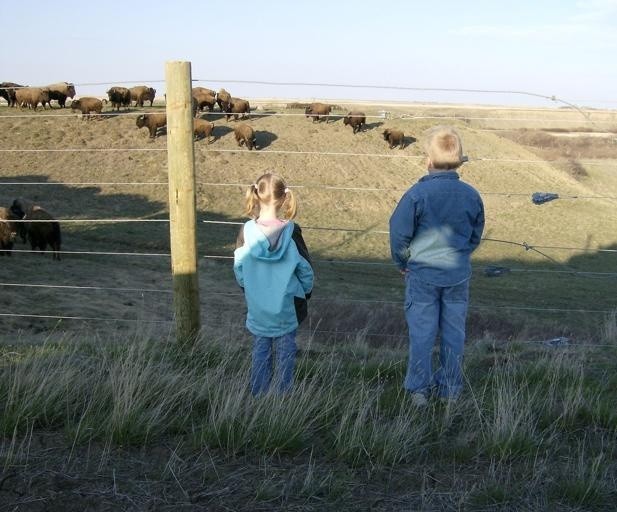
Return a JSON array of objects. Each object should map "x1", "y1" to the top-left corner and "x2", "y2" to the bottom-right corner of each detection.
[
  {"x1": 388, "y1": 123, "x2": 485, "y2": 406},
  {"x1": 233, "y1": 174, "x2": 314, "y2": 401},
  {"x1": 9, "y1": 199, "x2": 27, "y2": 244}
]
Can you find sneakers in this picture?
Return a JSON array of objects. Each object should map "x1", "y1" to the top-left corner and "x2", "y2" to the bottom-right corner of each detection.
[{"x1": 410, "y1": 392, "x2": 429, "y2": 407}]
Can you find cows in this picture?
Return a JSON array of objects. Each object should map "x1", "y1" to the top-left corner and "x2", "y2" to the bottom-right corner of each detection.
[
  {"x1": 136, "y1": 114, "x2": 167, "y2": 138},
  {"x1": 380, "y1": 128, "x2": 405, "y2": 150},
  {"x1": 192, "y1": 87, "x2": 250, "y2": 122},
  {"x1": 343, "y1": 111, "x2": 366, "y2": 134},
  {"x1": 71, "y1": 97, "x2": 107, "y2": 121},
  {"x1": 305, "y1": 103, "x2": 332, "y2": 123},
  {"x1": 0, "y1": 81, "x2": 76, "y2": 112},
  {"x1": 193, "y1": 117, "x2": 214, "y2": 145},
  {"x1": 107, "y1": 86, "x2": 156, "y2": 111},
  {"x1": 0, "y1": 199, "x2": 62, "y2": 261},
  {"x1": 234, "y1": 123, "x2": 256, "y2": 151}
]
